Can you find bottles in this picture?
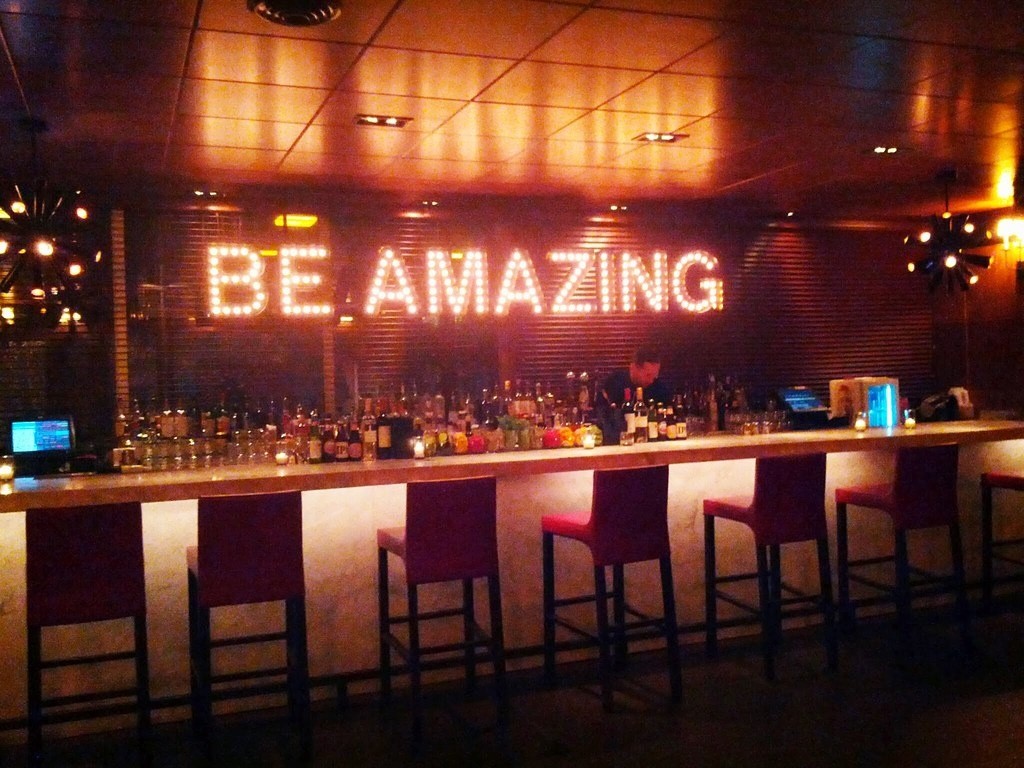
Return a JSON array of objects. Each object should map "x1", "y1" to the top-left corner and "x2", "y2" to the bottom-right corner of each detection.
[
  {"x1": 621, "y1": 375, "x2": 791, "y2": 445},
  {"x1": 112, "y1": 378, "x2": 589, "y2": 473}
]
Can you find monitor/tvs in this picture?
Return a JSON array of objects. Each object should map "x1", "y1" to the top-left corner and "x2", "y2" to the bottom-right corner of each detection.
[
  {"x1": 7, "y1": 414, "x2": 77, "y2": 458},
  {"x1": 774, "y1": 386, "x2": 829, "y2": 413}
]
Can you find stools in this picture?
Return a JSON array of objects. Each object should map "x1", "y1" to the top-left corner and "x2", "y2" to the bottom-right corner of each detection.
[
  {"x1": 704, "y1": 451, "x2": 840, "y2": 682},
  {"x1": 378, "y1": 475, "x2": 510, "y2": 747},
  {"x1": 26, "y1": 498, "x2": 152, "y2": 768},
  {"x1": 188, "y1": 489, "x2": 313, "y2": 767},
  {"x1": 540, "y1": 463, "x2": 684, "y2": 717},
  {"x1": 980, "y1": 471, "x2": 1024, "y2": 624},
  {"x1": 834, "y1": 442, "x2": 970, "y2": 680}
]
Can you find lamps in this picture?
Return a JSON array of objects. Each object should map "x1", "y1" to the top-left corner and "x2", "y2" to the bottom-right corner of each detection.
[
  {"x1": 902, "y1": 169, "x2": 994, "y2": 295},
  {"x1": 0, "y1": 177, "x2": 102, "y2": 326}
]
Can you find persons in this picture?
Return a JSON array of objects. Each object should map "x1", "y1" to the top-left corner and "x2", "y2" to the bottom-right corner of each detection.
[{"x1": 592, "y1": 348, "x2": 675, "y2": 420}]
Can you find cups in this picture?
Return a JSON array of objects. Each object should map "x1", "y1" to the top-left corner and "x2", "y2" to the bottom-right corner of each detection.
[
  {"x1": 619, "y1": 431, "x2": 634, "y2": 446},
  {"x1": 853, "y1": 411, "x2": 866, "y2": 432},
  {"x1": 582, "y1": 435, "x2": 596, "y2": 449},
  {"x1": 903, "y1": 409, "x2": 917, "y2": 429}
]
